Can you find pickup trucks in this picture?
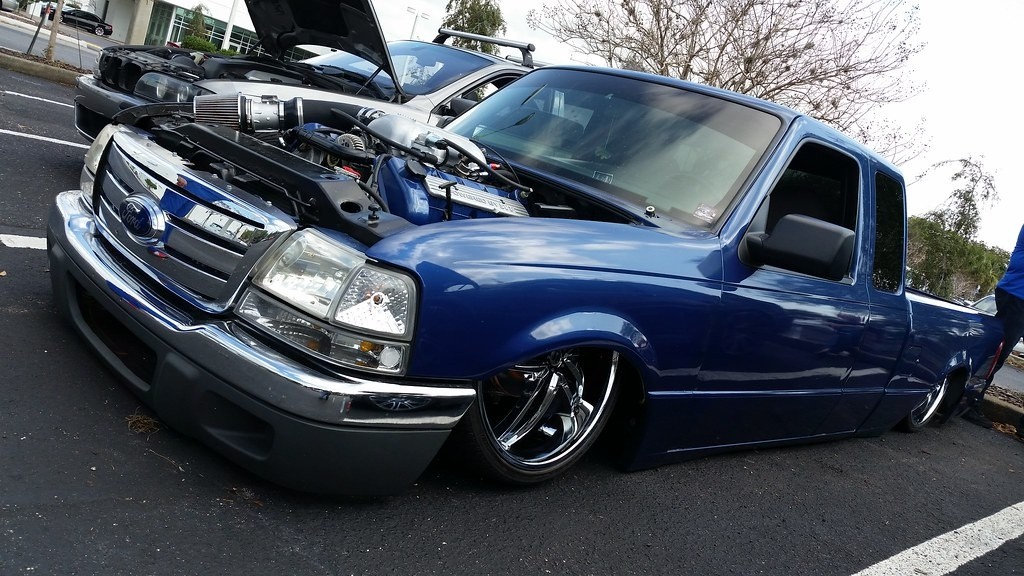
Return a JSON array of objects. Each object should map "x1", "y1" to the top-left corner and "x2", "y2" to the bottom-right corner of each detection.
[{"x1": 39, "y1": 64, "x2": 1010, "y2": 502}]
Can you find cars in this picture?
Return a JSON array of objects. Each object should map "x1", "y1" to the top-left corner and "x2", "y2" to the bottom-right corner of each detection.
[
  {"x1": 0, "y1": 1, "x2": 20, "y2": 13},
  {"x1": 963, "y1": 294, "x2": 1024, "y2": 359},
  {"x1": 49, "y1": 6, "x2": 115, "y2": 37}
]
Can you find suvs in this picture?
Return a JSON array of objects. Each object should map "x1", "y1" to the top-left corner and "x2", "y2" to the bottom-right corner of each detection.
[{"x1": 63, "y1": 0, "x2": 592, "y2": 148}]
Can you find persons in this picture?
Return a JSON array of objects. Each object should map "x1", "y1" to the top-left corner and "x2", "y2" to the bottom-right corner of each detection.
[{"x1": 957, "y1": 224, "x2": 1024, "y2": 429}]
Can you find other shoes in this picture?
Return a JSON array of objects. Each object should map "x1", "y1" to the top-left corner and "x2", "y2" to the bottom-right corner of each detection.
[{"x1": 965, "y1": 410, "x2": 994, "y2": 428}]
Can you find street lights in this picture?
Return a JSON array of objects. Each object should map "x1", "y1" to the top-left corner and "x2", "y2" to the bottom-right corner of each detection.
[{"x1": 398, "y1": 6, "x2": 430, "y2": 88}]
